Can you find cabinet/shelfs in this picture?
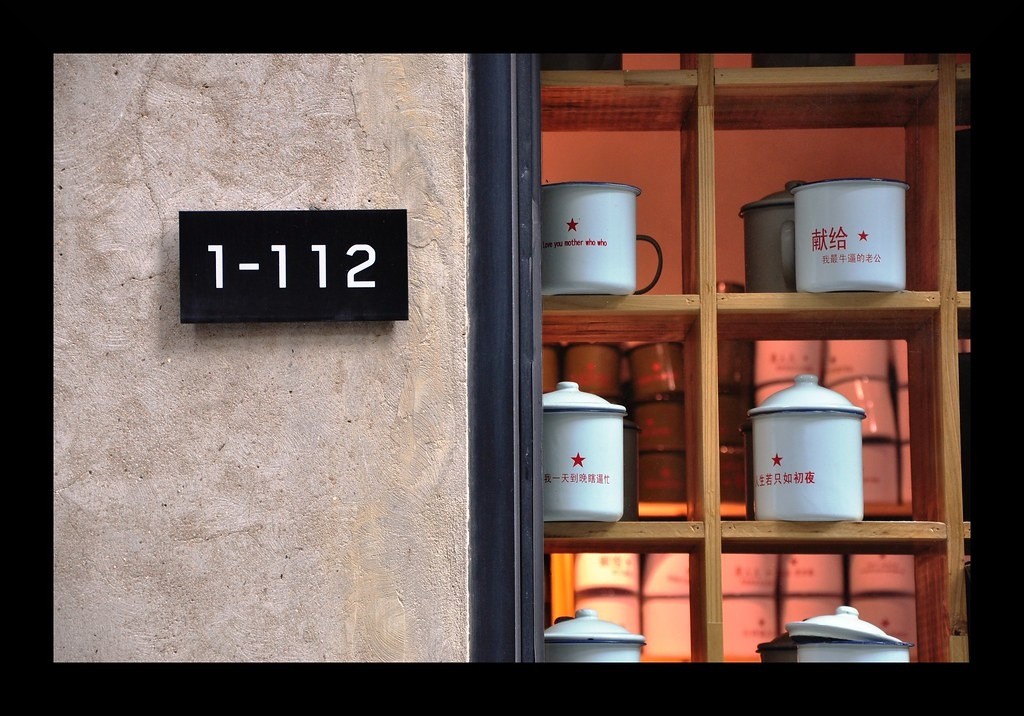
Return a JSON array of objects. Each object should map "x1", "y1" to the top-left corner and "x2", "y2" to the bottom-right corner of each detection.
[{"x1": 539, "y1": 53, "x2": 972, "y2": 663}]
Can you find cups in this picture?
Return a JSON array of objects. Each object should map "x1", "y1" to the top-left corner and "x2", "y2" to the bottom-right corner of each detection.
[
  {"x1": 539, "y1": 180, "x2": 663, "y2": 299},
  {"x1": 745, "y1": 339, "x2": 907, "y2": 523},
  {"x1": 791, "y1": 177, "x2": 910, "y2": 294},
  {"x1": 737, "y1": 178, "x2": 814, "y2": 293},
  {"x1": 542, "y1": 333, "x2": 754, "y2": 521},
  {"x1": 545, "y1": 552, "x2": 916, "y2": 662}
]
